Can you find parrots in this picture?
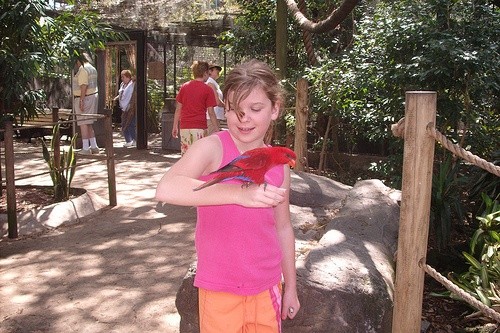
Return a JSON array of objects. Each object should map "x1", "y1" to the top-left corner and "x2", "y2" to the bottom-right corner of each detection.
[{"x1": 193, "y1": 146, "x2": 297, "y2": 191}]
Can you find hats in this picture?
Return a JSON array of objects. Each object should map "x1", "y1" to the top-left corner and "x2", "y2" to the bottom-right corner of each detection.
[{"x1": 208, "y1": 64, "x2": 222, "y2": 71}]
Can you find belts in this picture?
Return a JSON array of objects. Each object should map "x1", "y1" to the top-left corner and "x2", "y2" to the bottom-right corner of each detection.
[{"x1": 74, "y1": 92, "x2": 98, "y2": 98}]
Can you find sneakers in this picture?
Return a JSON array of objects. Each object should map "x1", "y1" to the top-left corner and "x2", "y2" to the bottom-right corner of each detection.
[
  {"x1": 77, "y1": 149, "x2": 92, "y2": 155},
  {"x1": 90, "y1": 147, "x2": 100, "y2": 154},
  {"x1": 123, "y1": 142, "x2": 132, "y2": 148},
  {"x1": 131, "y1": 140, "x2": 137, "y2": 146}
]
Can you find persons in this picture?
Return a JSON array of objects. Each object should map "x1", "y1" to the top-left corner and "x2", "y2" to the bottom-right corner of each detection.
[
  {"x1": 113, "y1": 69, "x2": 137, "y2": 147},
  {"x1": 72, "y1": 52, "x2": 99, "y2": 155},
  {"x1": 156, "y1": 59, "x2": 300, "y2": 333}
]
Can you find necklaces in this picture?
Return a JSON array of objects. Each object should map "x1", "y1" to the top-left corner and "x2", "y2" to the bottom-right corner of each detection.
[{"x1": 120, "y1": 79, "x2": 131, "y2": 100}]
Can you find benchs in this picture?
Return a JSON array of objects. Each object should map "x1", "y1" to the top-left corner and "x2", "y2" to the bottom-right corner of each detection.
[{"x1": 12, "y1": 108, "x2": 72, "y2": 143}]
